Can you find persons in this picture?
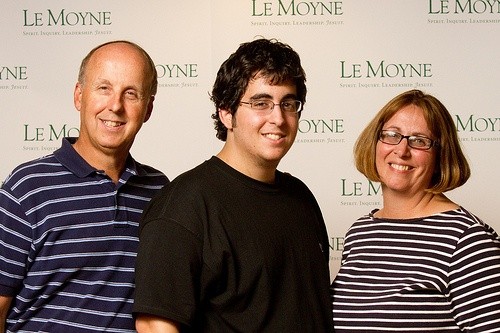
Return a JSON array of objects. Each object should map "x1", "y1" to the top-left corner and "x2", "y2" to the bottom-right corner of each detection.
[
  {"x1": 134, "y1": 35, "x2": 335, "y2": 333},
  {"x1": 0, "y1": 40, "x2": 170, "y2": 333},
  {"x1": 331, "y1": 89, "x2": 500, "y2": 333}
]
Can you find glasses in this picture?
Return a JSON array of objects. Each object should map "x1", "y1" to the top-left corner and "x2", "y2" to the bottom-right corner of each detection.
[
  {"x1": 377, "y1": 129, "x2": 440, "y2": 151},
  {"x1": 240, "y1": 99, "x2": 302, "y2": 113}
]
